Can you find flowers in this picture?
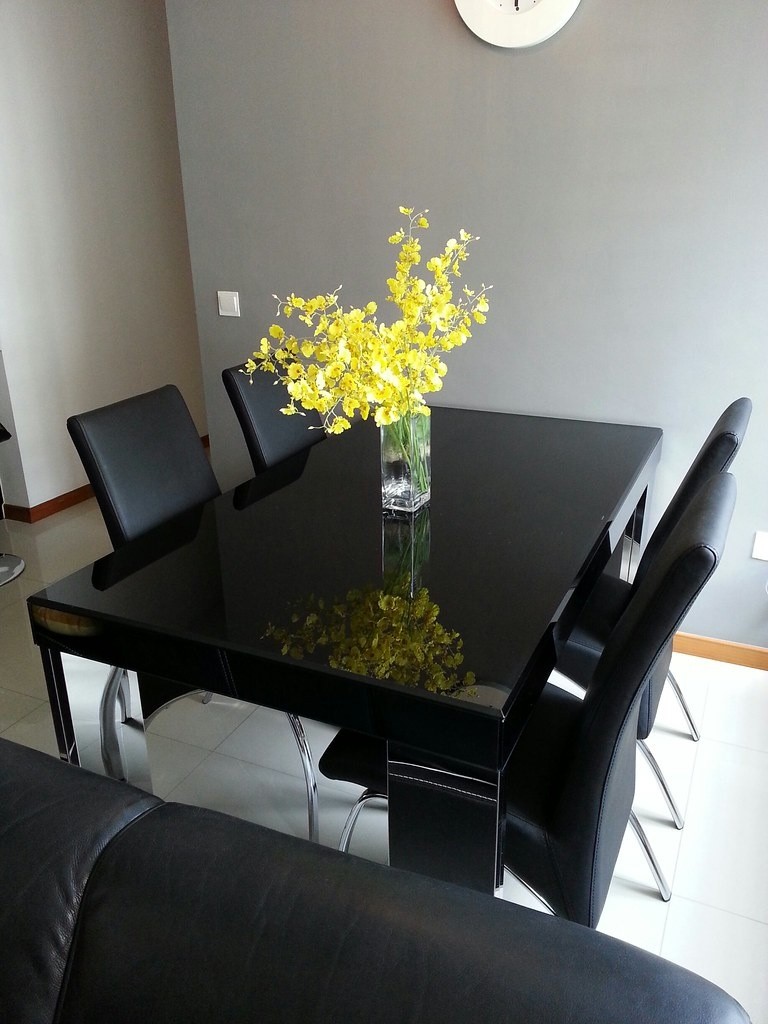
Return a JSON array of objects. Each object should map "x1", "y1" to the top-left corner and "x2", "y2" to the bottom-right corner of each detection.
[{"x1": 236, "y1": 207, "x2": 494, "y2": 434}]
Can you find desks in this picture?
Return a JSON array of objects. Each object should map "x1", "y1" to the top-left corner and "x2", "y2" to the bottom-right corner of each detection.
[{"x1": 25, "y1": 405, "x2": 664, "y2": 900}]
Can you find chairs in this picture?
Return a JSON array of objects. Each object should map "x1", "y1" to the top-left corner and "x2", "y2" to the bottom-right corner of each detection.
[
  {"x1": 221, "y1": 348, "x2": 327, "y2": 476},
  {"x1": 316, "y1": 472, "x2": 738, "y2": 930},
  {"x1": 554, "y1": 397, "x2": 753, "y2": 832},
  {"x1": 66, "y1": 383, "x2": 317, "y2": 841}
]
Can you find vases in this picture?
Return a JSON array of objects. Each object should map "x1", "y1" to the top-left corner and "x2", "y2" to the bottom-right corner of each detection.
[{"x1": 379, "y1": 408, "x2": 431, "y2": 520}]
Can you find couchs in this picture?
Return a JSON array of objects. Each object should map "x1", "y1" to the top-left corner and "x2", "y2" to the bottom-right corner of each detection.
[{"x1": 0, "y1": 738, "x2": 754, "y2": 1024}]
